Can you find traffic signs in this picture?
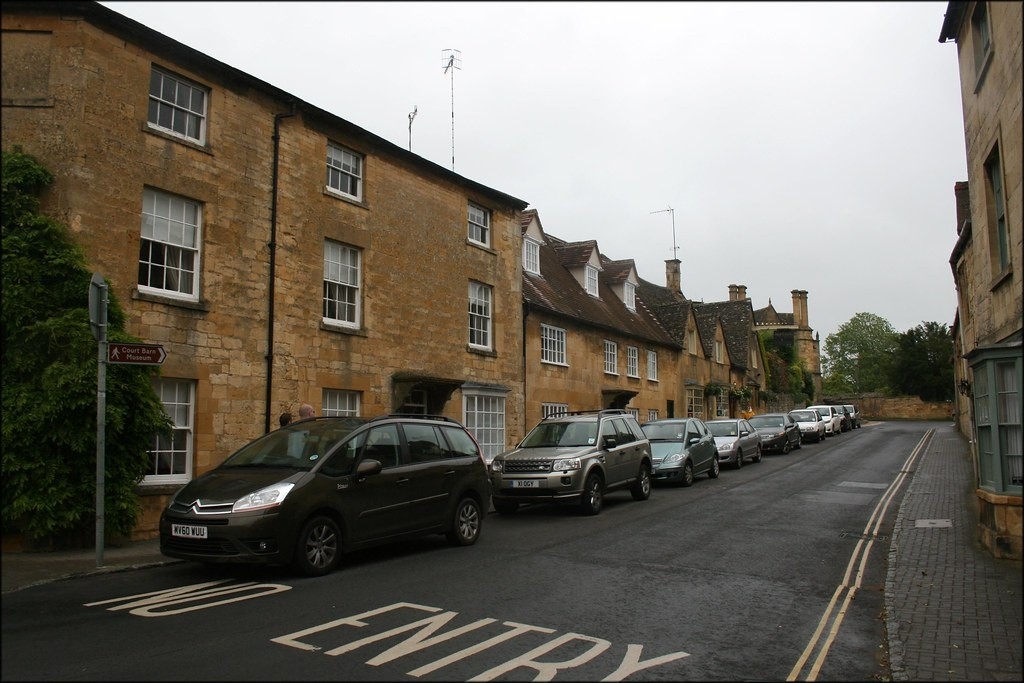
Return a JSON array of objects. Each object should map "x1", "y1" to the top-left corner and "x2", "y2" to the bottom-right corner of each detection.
[{"x1": 108, "y1": 341, "x2": 167, "y2": 365}]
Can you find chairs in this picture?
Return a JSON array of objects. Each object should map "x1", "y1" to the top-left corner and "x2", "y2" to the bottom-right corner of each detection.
[{"x1": 323, "y1": 439, "x2": 348, "y2": 468}]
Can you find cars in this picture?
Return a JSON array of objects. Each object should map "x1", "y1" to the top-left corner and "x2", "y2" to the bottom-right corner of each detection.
[
  {"x1": 788, "y1": 410, "x2": 826, "y2": 444},
  {"x1": 842, "y1": 405, "x2": 861, "y2": 429},
  {"x1": 638, "y1": 419, "x2": 721, "y2": 486},
  {"x1": 831, "y1": 406, "x2": 853, "y2": 432},
  {"x1": 808, "y1": 405, "x2": 842, "y2": 436},
  {"x1": 702, "y1": 419, "x2": 764, "y2": 470},
  {"x1": 747, "y1": 414, "x2": 803, "y2": 456},
  {"x1": 159, "y1": 415, "x2": 494, "y2": 573}
]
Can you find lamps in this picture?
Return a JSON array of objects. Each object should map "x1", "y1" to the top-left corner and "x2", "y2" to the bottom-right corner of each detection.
[{"x1": 958, "y1": 378, "x2": 971, "y2": 396}]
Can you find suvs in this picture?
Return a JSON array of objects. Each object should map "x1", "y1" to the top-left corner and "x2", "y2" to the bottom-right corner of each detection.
[{"x1": 489, "y1": 410, "x2": 653, "y2": 515}]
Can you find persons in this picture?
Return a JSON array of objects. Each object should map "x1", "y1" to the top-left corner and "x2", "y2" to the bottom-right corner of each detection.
[
  {"x1": 287, "y1": 404, "x2": 316, "y2": 459},
  {"x1": 268, "y1": 412, "x2": 293, "y2": 457},
  {"x1": 323, "y1": 439, "x2": 355, "y2": 476}
]
